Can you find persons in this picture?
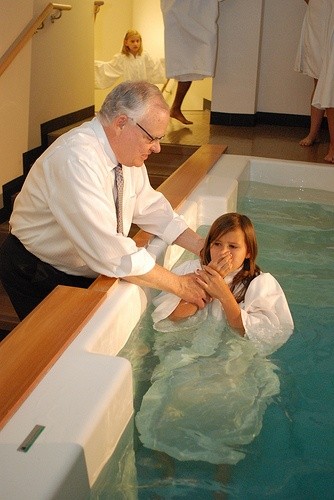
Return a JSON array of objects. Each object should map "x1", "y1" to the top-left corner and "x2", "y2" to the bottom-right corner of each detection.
[
  {"x1": 296, "y1": 0, "x2": 334, "y2": 163},
  {"x1": 95, "y1": 29, "x2": 167, "y2": 90},
  {"x1": 0, "y1": 81, "x2": 213, "y2": 321},
  {"x1": 160, "y1": 0, "x2": 220, "y2": 125},
  {"x1": 155, "y1": 213, "x2": 294, "y2": 334}
]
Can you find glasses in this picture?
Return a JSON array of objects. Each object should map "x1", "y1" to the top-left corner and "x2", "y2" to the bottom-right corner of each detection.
[{"x1": 127, "y1": 116, "x2": 165, "y2": 144}]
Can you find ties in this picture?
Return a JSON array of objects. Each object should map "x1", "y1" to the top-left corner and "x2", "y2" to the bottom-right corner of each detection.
[{"x1": 114, "y1": 163, "x2": 124, "y2": 236}]
